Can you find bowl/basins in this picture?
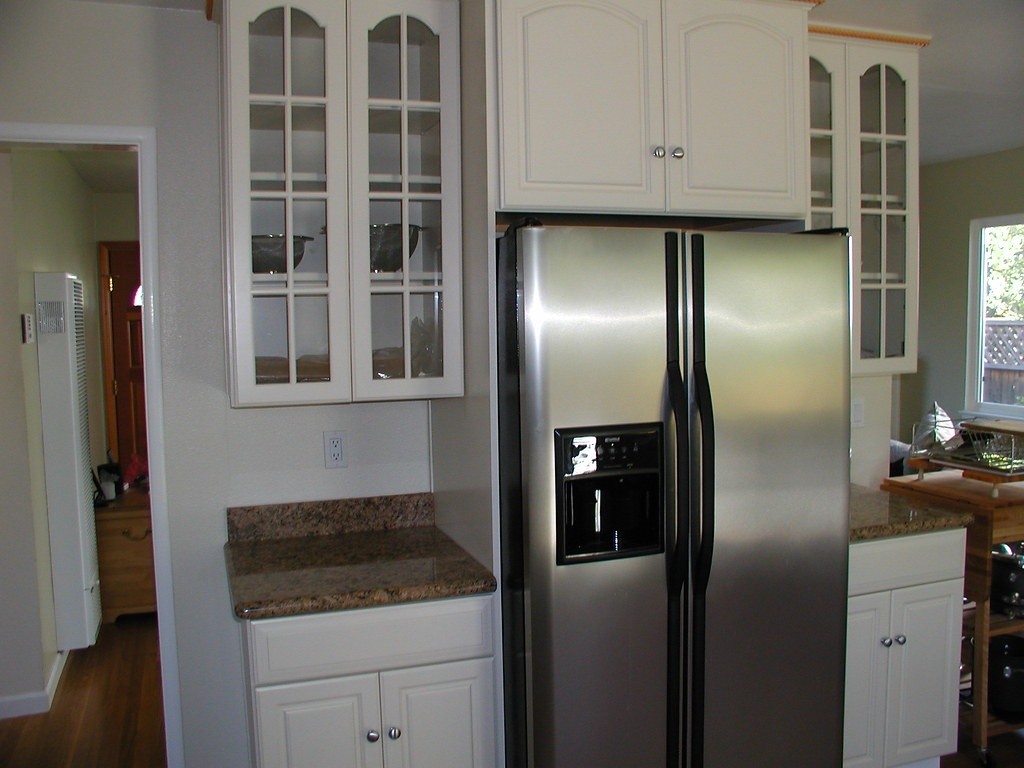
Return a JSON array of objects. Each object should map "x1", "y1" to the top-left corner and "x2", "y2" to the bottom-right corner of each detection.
[
  {"x1": 373, "y1": 224, "x2": 423, "y2": 274},
  {"x1": 252, "y1": 235, "x2": 314, "y2": 273}
]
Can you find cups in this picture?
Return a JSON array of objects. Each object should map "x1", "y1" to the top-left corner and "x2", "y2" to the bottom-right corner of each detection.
[{"x1": 101, "y1": 484, "x2": 115, "y2": 499}]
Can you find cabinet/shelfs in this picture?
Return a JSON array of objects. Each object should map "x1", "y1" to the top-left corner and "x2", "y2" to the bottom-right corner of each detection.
[
  {"x1": 221, "y1": 0, "x2": 465, "y2": 410},
  {"x1": 485, "y1": 0, "x2": 825, "y2": 218},
  {"x1": 809, "y1": 22, "x2": 931, "y2": 376},
  {"x1": 842, "y1": 469, "x2": 1024, "y2": 768},
  {"x1": 244, "y1": 592, "x2": 496, "y2": 768},
  {"x1": 95, "y1": 486, "x2": 157, "y2": 624}
]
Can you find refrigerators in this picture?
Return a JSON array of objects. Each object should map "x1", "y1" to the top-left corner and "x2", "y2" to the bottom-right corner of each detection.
[{"x1": 499, "y1": 226, "x2": 852, "y2": 768}]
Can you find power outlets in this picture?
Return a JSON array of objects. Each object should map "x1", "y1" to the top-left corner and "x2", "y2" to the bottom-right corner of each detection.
[{"x1": 322, "y1": 430, "x2": 349, "y2": 468}]
